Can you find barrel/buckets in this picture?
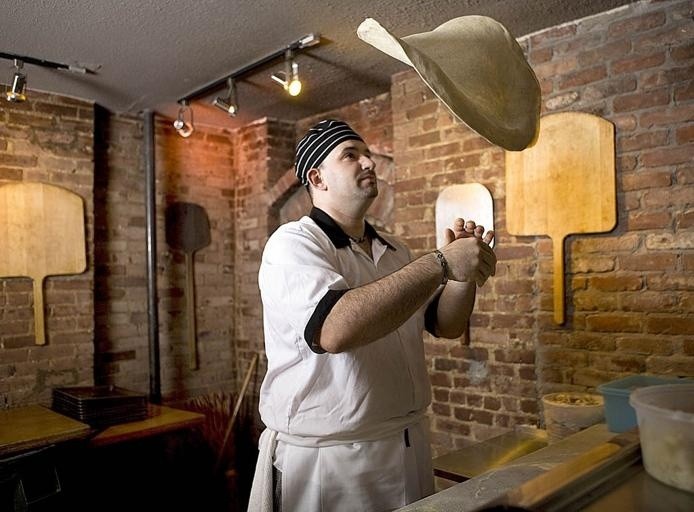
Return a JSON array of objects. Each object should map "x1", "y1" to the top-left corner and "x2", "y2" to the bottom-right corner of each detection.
[{"x1": 542, "y1": 392, "x2": 606, "y2": 446}]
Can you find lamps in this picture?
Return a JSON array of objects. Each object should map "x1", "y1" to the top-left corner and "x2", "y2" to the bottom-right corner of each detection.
[
  {"x1": 5, "y1": 59, "x2": 27, "y2": 102},
  {"x1": 172, "y1": 52, "x2": 302, "y2": 137}
]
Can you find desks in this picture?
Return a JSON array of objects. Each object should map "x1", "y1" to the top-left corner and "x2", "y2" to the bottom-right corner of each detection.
[{"x1": 0, "y1": 401, "x2": 206, "y2": 512}]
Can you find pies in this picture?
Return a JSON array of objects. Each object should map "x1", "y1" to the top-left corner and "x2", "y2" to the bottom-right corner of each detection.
[{"x1": 356, "y1": 15, "x2": 542, "y2": 151}]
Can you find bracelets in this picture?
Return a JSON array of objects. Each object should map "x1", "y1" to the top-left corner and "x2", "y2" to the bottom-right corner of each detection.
[{"x1": 433, "y1": 249, "x2": 449, "y2": 286}]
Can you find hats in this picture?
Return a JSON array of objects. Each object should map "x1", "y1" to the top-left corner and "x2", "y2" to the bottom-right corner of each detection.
[{"x1": 294, "y1": 115, "x2": 363, "y2": 187}]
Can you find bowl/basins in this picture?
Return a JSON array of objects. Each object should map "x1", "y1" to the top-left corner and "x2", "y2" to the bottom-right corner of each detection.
[
  {"x1": 542, "y1": 391, "x2": 604, "y2": 415},
  {"x1": 628, "y1": 382, "x2": 694, "y2": 496}
]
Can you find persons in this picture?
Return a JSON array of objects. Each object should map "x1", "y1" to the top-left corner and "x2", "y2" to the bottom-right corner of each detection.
[{"x1": 246, "y1": 119, "x2": 498, "y2": 511}]
